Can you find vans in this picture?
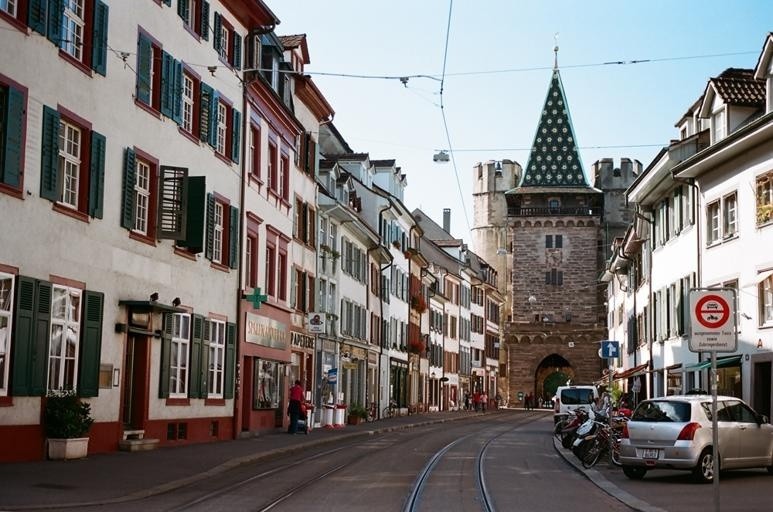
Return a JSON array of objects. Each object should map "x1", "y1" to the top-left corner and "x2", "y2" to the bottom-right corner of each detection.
[{"x1": 554, "y1": 385, "x2": 601, "y2": 417}]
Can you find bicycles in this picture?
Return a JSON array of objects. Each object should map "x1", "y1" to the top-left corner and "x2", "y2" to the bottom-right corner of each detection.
[{"x1": 382, "y1": 399, "x2": 398, "y2": 420}]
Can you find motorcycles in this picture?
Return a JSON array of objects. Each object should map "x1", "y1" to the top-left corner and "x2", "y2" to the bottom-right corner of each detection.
[{"x1": 552, "y1": 406, "x2": 634, "y2": 470}]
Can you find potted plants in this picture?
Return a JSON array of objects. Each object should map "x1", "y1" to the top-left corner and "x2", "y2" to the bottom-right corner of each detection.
[
  {"x1": 349, "y1": 403, "x2": 368, "y2": 425},
  {"x1": 46, "y1": 387, "x2": 96, "y2": 461}
]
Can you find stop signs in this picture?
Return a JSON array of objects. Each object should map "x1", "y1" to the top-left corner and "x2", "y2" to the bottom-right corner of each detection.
[{"x1": 689, "y1": 290, "x2": 736, "y2": 351}]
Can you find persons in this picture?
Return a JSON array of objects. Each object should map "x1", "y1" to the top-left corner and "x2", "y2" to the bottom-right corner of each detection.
[
  {"x1": 465, "y1": 391, "x2": 472, "y2": 411},
  {"x1": 529, "y1": 392, "x2": 533, "y2": 411},
  {"x1": 524, "y1": 394, "x2": 529, "y2": 411},
  {"x1": 537, "y1": 392, "x2": 551, "y2": 409},
  {"x1": 473, "y1": 390, "x2": 480, "y2": 413},
  {"x1": 289, "y1": 379, "x2": 304, "y2": 429},
  {"x1": 480, "y1": 392, "x2": 488, "y2": 412}
]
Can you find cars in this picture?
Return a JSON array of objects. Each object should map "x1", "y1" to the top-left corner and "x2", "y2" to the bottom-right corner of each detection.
[{"x1": 615, "y1": 394, "x2": 773, "y2": 484}]
